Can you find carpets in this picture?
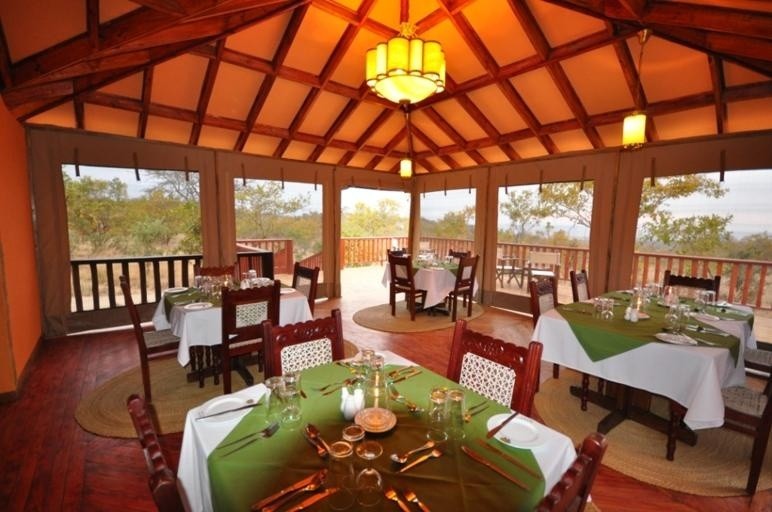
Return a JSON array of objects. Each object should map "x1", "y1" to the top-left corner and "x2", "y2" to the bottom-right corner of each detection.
[
  {"x1": 533, "y1": 371, "x2": 772, "y2": 497},
  {"x1": 352, "y1": 297, "x2": 484, "y2": 333},
  {"x1": 74, "y1": 337, "x2": 359, "y2": 439}
]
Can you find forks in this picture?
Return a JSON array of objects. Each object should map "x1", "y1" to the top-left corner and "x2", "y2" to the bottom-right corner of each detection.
[
  {"x1": 401, "y1": 485, "x2": 429, "y2": 512},
  {"x1": 221, "y1": 425, "x2": 280, "y2": 459},
  {"x1": 216, "y1": 421, "x2": 277, "y2": 451},
  {"x1": 383, "y1": 482, "x2": 409, "y2": 511}
]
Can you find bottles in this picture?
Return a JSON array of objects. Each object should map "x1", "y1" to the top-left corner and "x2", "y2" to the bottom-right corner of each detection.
[
  {"x1": 625, "y1": 307, "x2": 632, "y2": 320},
  {"x1": 341, "y1": 387, "x2": 350, "y2": 412},
  {"x1": 630, "y1": 309, "x2": 638, "y2": 323},
  {"x1": 343, "y1": 395, "x2": 356, "y2": 421},
  {"x1": 354, "y1": 388, "x2": 366, "y2": 410}
]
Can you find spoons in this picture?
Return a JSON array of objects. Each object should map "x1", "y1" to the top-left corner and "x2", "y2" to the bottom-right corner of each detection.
[
  {"x1": 307, "y1": 423, "x2": 331, "y2": 453},
  {"x1": 390, "y1": 441, "x2": 435, "y2": 463},
  {"x1": 400, "y1": 442, "x2": 446, "y2": 474},
  {"x1": 303, "y1": 430, "x2": 329, "y2": 459}
]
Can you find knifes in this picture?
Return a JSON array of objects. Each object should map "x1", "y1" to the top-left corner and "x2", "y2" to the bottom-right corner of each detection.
[
  {"x1": 475, "y1": 437, "x2": 545, "y2": 482},
  {"x1": 486, "y1": 410, "x2": 520, "y2": 440},
  {"x1": 461, "y1": 445, "x2": 529, "y2": 492},
  {"x1": 196, "y1": 402, "x2": 264, "y2": 423},
  {"x1": 388, "y1": 369, "x2": 423, "y2": 386},
  {"x1": 287, "y1": 487, "x2": 341, "y2": 512},
  {"x1": 251, "y1": 472, "x2": 318, "y2": 511}
]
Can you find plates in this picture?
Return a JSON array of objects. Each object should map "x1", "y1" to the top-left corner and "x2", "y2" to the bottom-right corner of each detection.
[
  {"x1": 655, "y1": 332, "x2": 694, "y2": 345},
  {"x1": 165, "y1": 287, "x2": 188, "y2": 293},
  {"x1": 355, "y1": 407, "x2": 397, "y2": 433},
  {"x1": 352, "y1": 352, "x2": 388, "y2": 368},
  {"x1": 184, "y1": 302, "x2": 213, "y2": 310},
  {"x1": 487, "y1": 413, "x2": 541, "y2": 449},
  {"x1": 201, "y1": 395, "x2": 249, "y2": 422}
]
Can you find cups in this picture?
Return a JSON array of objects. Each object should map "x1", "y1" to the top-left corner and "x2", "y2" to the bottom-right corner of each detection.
[
  {"x1": 356, "y1": 441, "x2": 383, "y2": 507},
  {"x1": 362, "y1": 349, "x2": 374, "y2": 364},
  {"x1": 707, "y1": 290, "x2": 715, "y2": 305},
  {"x1": 370, "y1": 356, "x2": 384, "y2": 374},
  {"x1": 264, "y1": 376, "x2": 286, "y2": 424},
  {"x1": 327, "y1": 441, "x2": 356, "y2": 511},
  {"x1": 341, "y1": 425, "x2": 368, "y2": 490},
  {"x1": 428, "y1": 386, "x2": 450, "y2": 430},
  {"x1": 284, "y1": 374, "x2": 302, "y2": 412},
  {"x1": 426, "y1": 393, "x2": 448, "y2": 442},
  {"x1": 593, "y1": 297, "x2": 603, "y2": 320},
  {"x1": 677, "y1": 305, "x2": 690, "y2": 324},
  {"x1": 603, "y1": 299, "x2": 614, "y2": 319},
  {"x1": 446, "y1": 387, "x2": 465, "y2": 441},
  {"x1": 281, "y1": 388, "x2": 302, "y2": 429}
]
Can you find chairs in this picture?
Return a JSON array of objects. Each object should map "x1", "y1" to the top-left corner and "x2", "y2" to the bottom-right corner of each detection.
[
  {"x1": 432, "y1": 254, "x2": 480, "y2": 321},
  {"x1": 387, "y1": 247, "x2": 406, "y2": 264},
  {"x1": 263, "y1": 307, "x2": 345, "y2": 381},
  {"x1": 447, "y1": 247, "x2": 471, "y2": 308},
  {"x1": 534, "y1": 431, "x2": 609, "y2": 512},
  {"x1": 665, "y1": 373, "x2": 772, "y2": 492},
  {"x1": 743, "y1": 346, "x2": 772, "y2": 381},
  {"x1": 529, "y1": 278, "x2": 565, "y2": 379},
  {"x1": 663, "y1": 270, "x2": 721, "y2": 301},
  {"x1": 570, "y1": 269, "x2": 590, "y2": 302},
  {"x1": 119, "y1": 275, "x2": 182, "y2": 402},
  {"x1": 126, "y1": 392, "x2": 185, "y2": 511},
  {"x1": 447, "y1": 318, "x2": 544, "y2": 418},
  {"x1": 387, "y1": 253, "x2": 431, "y2": 321},
  {"x1": 210, "y1": 280, "x2": 281, "y2": 395},
  {"x1": 193, "y1": 260, "x2": 238, "y2": 282},
  {"x1": 291, "y1": 262, "x2": 320, "y2": 317}
]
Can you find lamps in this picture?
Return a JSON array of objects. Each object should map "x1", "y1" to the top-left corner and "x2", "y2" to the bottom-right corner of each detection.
[
  {"x1": 365, "y1": 22, "x2": 446, "y2": 114},
  {"x1": 619, "y1": 28, "x2": 651, "y2": 153},
  {"x1": 400, "y1": 159, "x2": 412, "y2": 178}
]
